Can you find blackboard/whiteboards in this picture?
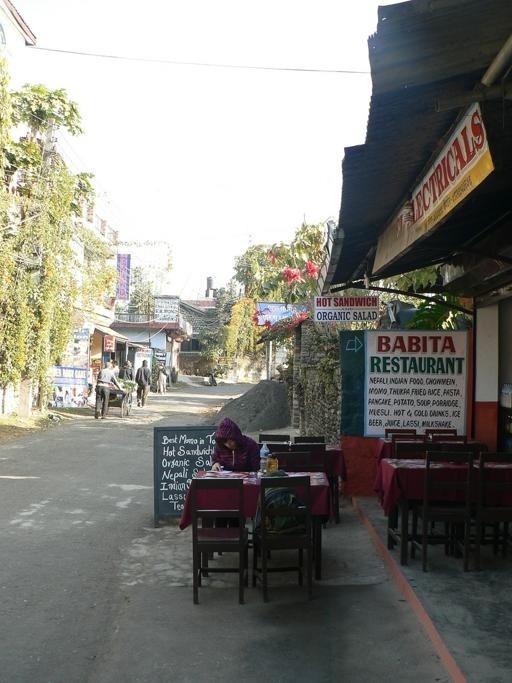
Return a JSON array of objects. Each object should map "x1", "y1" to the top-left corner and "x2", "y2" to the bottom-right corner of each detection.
[{"x1": 154, "y1": 426, "x2": 218, "y2": 516}]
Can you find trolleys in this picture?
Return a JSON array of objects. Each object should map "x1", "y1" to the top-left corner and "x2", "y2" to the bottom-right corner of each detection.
[{"x1": 108, "y1": 388, "x2": 132, "y2": 417}]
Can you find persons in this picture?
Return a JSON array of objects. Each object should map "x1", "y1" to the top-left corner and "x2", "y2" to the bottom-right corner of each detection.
[
  {"x1": 210, "y1": 417, "x2": 261, "y2": 528},
  {"x1": 95, "y1": 359, "x2": 167, "y2": 419}
]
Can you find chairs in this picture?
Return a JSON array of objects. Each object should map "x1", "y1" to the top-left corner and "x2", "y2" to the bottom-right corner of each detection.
[
  {"x1": 373, "y1": 428, "x2": 512, "y2": 572},
  {"x1": 181, "y1": 434, "x2": 347, "y2": 605}
]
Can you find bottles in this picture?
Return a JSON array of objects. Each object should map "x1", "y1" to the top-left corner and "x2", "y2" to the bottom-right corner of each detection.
[{"x1": 260, "y1": 443, "x2": 279, "y2": 473}]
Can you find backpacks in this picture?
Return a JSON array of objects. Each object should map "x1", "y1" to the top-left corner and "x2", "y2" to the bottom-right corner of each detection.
[{"x1": 256, "y1": 469, "x2": 309, "y2": 537}]
